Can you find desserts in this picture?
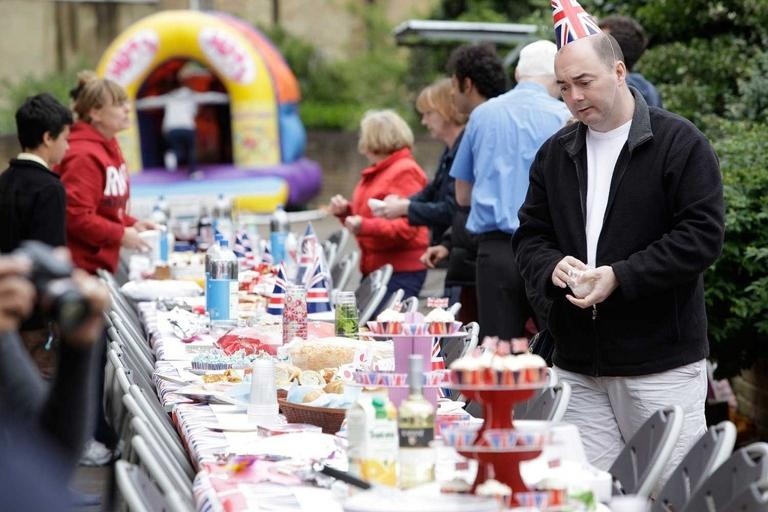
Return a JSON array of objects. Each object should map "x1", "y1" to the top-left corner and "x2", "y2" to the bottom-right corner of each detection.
[{"x1": 349, "y1": 289, "x2": 570, "y2": 512}]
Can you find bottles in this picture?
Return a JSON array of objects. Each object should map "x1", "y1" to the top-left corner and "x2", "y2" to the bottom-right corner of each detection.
[
  {"x1": 395, "y1": 352, "x2": 438, "y2": 492},
  {"x1": 147, "y1": 189, "x2": 296, "y2": 325}
]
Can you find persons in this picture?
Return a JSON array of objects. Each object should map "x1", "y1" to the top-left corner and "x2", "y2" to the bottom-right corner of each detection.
[
  {"x1": 598, "y1": 15, "x2": 662, "y2": 107},
  {"x1": 54, "y1": 74, "x2": 157, "y2": 466},
  {"x1": 328, "y1": 110, "x2": 430, "y2": 320},
  {"x1": 513, "y1": 32, "x2": 721, "y2": 494},
  {"x1": 133, "y1": 66, "x2": 230, "y2": 182},
  {"x1": 367, "y1": 80, "x2": 467, "y2": 327},
  {"x1": 0, "y1": 94, "x2": 74, "y2": 251},
  {"x1": 0, "y1": 238, "x2": 108, "y2": 512},
  {"x1": 418, "y1": 44, "x2": 506, "y2": 325},
  {"x1": 447, "y1": 40, "x2": 574, "y2": 339}
]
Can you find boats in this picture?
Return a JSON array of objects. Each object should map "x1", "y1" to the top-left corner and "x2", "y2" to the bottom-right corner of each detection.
[{"x1": 93, "y1": 7, "x2": 327, "y2": 221}]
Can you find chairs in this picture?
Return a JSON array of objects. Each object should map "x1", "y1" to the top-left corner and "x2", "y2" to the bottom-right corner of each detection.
[{"x1": 98, "y1": 225, "x2": 768, "y2": 512}]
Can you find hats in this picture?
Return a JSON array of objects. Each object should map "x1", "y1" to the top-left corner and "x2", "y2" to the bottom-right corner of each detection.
[{"x1": 549, "y1": 0, "x2": 604, "y2": 50}]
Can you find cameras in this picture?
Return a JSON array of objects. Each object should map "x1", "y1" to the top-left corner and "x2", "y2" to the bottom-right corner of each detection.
[{"x1": 9, "y1": 237, "x2": 91, "y2": 334}]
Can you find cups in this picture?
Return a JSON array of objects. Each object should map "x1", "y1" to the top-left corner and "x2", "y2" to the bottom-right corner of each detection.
[
  {"x1": 244, "y1": 357, "x2": 280, "y2": 424},
  {"x1": 280, "y1": 284, "x2": 308, "y2": 344},
  {"x1": 332, "y1": 291, "x2": 359, "y2": 340}
]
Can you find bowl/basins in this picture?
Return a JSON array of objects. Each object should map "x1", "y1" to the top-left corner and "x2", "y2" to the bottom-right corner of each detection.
[{"x1": 366, "y1": 199, "x2": 386, "y2": 218}]
